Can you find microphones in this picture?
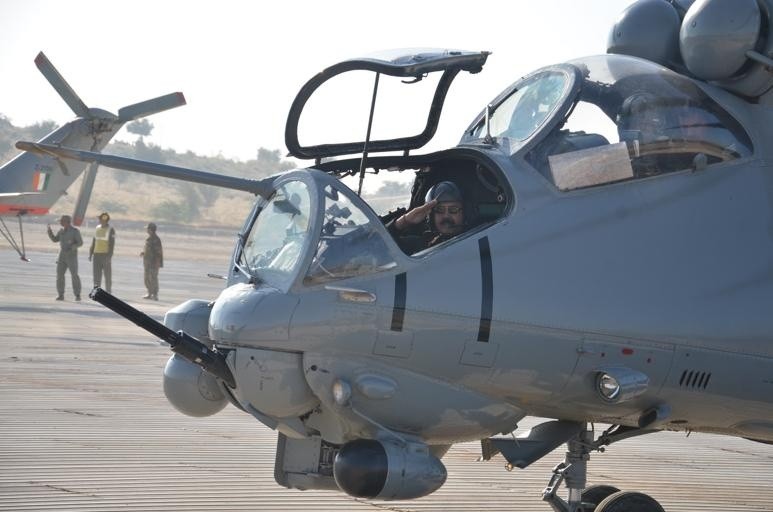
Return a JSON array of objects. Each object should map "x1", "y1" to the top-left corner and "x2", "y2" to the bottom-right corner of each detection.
[{"x1": 448, "y1": 224, "x2": 464, "y2": 228}]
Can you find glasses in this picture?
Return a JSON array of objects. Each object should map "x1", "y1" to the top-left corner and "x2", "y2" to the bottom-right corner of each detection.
[{"x1": 433, "y1": 206, "x2": 462, "y2": 213}]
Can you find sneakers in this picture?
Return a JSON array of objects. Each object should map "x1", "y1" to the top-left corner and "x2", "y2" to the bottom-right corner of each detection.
[
  {"x1": 143, "y1": 293, "x2": 159, "y2": 301},
  {"x1": 56, "y1": 294, "x2": 64, "y2": 300},
  {"x1": 75, "y1": 295, "x2": 81, "y2": 301}
]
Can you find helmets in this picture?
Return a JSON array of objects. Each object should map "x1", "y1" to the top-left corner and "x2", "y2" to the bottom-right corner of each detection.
[{"x1": 425, "y1": 180, "x2": 474, "y2": 229}]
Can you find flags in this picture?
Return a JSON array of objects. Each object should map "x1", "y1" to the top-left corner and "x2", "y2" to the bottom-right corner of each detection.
[{"x1": 33, "y1": 171, "x2": 50, "y2": 192}]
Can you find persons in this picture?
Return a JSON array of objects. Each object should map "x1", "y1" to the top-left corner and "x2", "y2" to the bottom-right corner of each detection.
[
  {"x1": 368, "y1": 181, "x2": 469, "y2": 255},
  {"x1": 47, "y1": 215, "x2": 83, "y2": 301},
  {"x1": 140, "y1": 223, "x2": 163, "y2": 300},
  {"x1": 88, "y1": 212, "x2": 115, "y2": 294}
]
